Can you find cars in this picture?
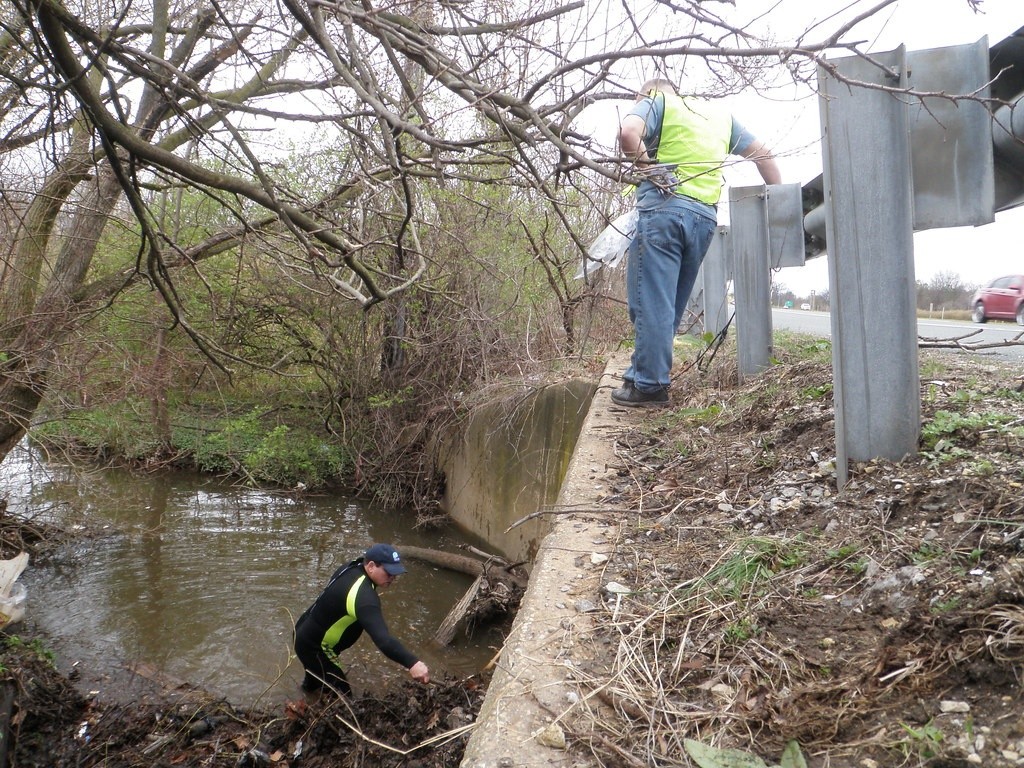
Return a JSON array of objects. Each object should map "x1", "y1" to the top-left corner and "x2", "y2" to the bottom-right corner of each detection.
[
  {"x1": 801, "y1": 303, "x2": 811, "y2": 311},
  {"x1": 971, "y1": 276, "x2": 1024, "y2": 326}
]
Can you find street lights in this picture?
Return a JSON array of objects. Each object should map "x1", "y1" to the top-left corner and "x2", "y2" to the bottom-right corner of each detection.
[{"x1": 811, "y1": 290, "x2": 815, "y2": 310}]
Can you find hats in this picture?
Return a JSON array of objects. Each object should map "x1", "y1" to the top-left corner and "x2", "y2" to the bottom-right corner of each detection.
[{"x1": 367, "y1": 544, "x2": 408, "y2": 575}]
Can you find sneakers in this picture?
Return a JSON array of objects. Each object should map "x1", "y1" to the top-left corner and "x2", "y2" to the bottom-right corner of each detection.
[
  {"x1": 611, "y1": 385, "x2": 671, "y2": 407},
  {"x1": 622, "y1": 380, "x2": 634, "y2": 389}
]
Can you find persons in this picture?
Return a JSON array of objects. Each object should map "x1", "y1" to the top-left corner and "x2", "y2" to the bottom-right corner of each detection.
[
  {"x1": 293, "y1": 544, "x2": 429, "y2": 699},
  {"x1": 611, "y1": 79, "x2": 781, "y2": 409}
]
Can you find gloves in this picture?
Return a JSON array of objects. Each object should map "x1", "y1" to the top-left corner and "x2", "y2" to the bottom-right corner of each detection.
[{"x1": 649, "y1": 164, "x2": 680, "y2": 196}]
